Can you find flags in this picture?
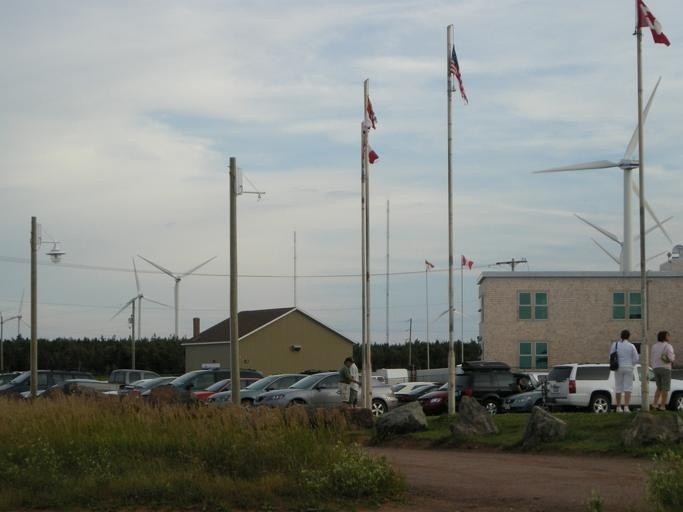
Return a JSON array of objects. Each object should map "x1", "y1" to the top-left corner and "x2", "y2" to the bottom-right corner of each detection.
[
  {"x1": 462, "y1": 256, "x2": 474, "y2": 270},
  {"x1": 448, "y1": 45, "x2": 469, "y2": 105},
  {"x1": 368, "y1": 147, "x2": 378, "y2": 164},
  {"x1": 366, "y1": 96, "x2": 378, "y2": 130},
  {"x1": 425, "y1": 260, "x2": 434, "y2": 270},
  {"x1": 635, "y1": 0, "x2": 671, "y2": 48}
]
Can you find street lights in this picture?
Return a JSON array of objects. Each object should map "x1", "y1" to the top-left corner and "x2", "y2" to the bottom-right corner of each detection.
[{"x1": 29, "y1": 214, "x2": 64, "y2": 398}]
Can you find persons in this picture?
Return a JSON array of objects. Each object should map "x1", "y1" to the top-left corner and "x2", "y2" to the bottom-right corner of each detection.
[
  {"x1": 344, "y1": 357, "x2": 360, "y2": 409},
  {"x1": 649, "y1": 330, "x2": 675, "y2": 411},
  {"x1": 338, "y1": 360, "x2": 361, "y2": 404},
  {"x1": 608, "y1": 329, "x2": 640, "y2": 413}
]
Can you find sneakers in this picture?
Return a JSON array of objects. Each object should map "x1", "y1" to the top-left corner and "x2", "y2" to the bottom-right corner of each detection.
[
  {"x1": 615, "y1": 406, "x2": 623, "y2": 412},
  {"x1": 650, "y1": 403, "x2": 666, "y2": 411},
  {"x1": 623, "y1": 407, "x2": 632, "y2": 413}
]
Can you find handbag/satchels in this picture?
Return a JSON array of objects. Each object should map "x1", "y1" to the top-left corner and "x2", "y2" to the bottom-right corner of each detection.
[
  {"x1": 610, "y1": 351, "x2": 619, "y2": 371},
  {"x1": 661, "y1": 353, "x2": 671, "y2": 363}
]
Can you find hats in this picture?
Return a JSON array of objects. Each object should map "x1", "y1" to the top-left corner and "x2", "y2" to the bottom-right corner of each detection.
[{"x1": 345, "y1": 356, "x2": 355, "y2": 363}]
